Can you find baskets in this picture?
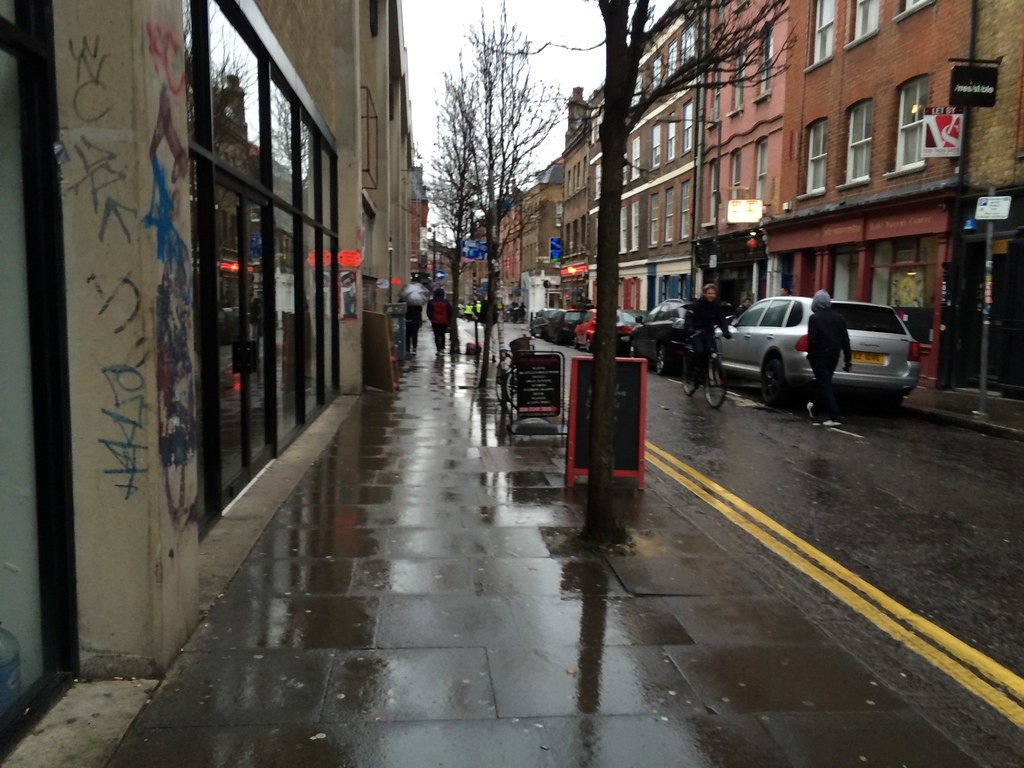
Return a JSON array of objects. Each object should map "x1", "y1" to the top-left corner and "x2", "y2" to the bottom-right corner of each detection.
[{"x1": 509, "y1": 337, "x2": 532, "y2": 354}]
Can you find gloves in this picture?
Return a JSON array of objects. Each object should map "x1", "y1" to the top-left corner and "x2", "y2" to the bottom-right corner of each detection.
[{"x1": 723, "y1": 331, "x2": 731, "y2": 339}]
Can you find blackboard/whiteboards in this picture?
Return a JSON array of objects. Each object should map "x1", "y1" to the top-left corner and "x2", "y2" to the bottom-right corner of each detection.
[
  {"x1": 515, "y1": 354, "x2": 561, "y2": 418},
  {"x1": 568, "y1": 355, "x2": 648, "y2": 476}
]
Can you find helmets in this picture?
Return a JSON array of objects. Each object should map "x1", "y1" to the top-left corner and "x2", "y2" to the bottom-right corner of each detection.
[{"x1": 477, "y1": 301, "x2": 480, "y2": 304}]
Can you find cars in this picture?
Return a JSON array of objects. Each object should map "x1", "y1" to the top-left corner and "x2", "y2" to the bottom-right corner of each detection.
[
  {"x1": 573, "y1": 310, "x2": 639, "y2": 352},
  {"x1": 218, "y1": 287, "x2": 240, "y2": 334},
  {"x1": 528, "y1": 309, "x2": 582, "y2": 345}
]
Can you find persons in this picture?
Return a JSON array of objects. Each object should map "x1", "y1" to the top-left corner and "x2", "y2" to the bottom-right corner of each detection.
[
  {"x1": 458, "y1": 300, "x2": 527, "y2": 323},
  {"x1": 735, "y1": 298, "x2": 752, "y2": 326},
  {"x1": 779, "y1": 286, "x2": 792, "y2": 296},
  {"x1": 398, "y1": 299, "x2": 423, "y2": 355},
  {"x1": 684, "y1": 283, "x2": 731, "y2": 384},
  {"x1": 807, "y1": 288, "x2": 853, "y2": 426},
  {"x1": 427, "y1": 288, "x2": 453, "y2": 356}
]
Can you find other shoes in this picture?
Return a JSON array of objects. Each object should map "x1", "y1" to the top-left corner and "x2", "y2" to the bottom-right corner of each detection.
[
  {"x1": 436, "y1": 349, "x2": 444, "y2": 356},
  {"x1": 406, "y1": 347, "x2": 416, "y2": 355},
  {"x1": 822, "y1": 419, "x2": 841, "y2": 426},
  {"x1": 807, "y1": 402, "x2": 816, "y2": 417}
]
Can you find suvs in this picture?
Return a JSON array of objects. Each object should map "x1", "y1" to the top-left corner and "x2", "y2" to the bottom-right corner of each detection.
[
  {"x1": 712, "y1": 297, "x2": 920, "y2": 417},
  {"x1": 631, "y1": 297, "x2": 707, "y2": 377}
]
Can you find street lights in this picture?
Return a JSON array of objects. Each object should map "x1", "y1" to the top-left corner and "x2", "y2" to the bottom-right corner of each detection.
[{"x1": 655, "y1": 116, "x2": 722, "y2": 295}]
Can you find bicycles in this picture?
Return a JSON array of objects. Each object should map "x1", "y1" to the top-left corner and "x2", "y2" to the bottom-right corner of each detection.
[{"x1": 681, "y1": 328, "x2": 731, "y2": 408}]
